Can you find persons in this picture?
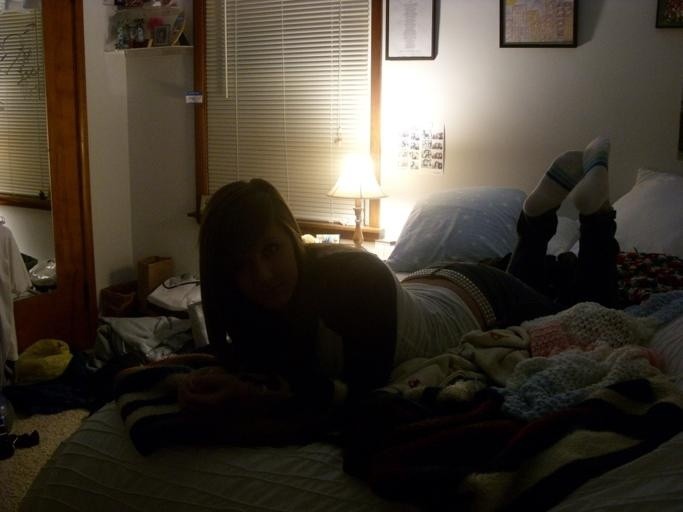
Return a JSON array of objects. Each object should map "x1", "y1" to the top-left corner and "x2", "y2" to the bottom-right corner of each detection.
[
  {"x1": 176, "y1": 134, "x2": 622, "y2": 404},
  {"x1": 128, "y1": 24, "x2": 149, "y2": 47}
]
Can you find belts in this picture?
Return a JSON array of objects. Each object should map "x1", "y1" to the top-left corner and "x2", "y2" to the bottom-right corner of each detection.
[{"x1": 400, "y1": 269, "x2": 496, "y2": 326}]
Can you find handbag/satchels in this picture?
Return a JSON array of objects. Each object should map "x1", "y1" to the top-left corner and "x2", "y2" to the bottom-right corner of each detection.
[{"x1": 138, "y1": 256, "x2": 176, "y2": 310}]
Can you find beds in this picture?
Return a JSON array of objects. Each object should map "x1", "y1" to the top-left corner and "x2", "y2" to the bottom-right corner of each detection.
[{"x1": 18, "y1": 297, "x2": 681, "y2": 512}]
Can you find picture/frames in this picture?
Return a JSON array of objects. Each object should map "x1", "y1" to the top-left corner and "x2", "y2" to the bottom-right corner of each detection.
[
  {"x1": 385, "y1": 1, "x2": 435, "y2": 59},
  {"x1": 653, "y1": 0, "x2": 682, "y2": 30},
  {"x1": 499, "y1": 0, "x2": 577, "y2": 50}
]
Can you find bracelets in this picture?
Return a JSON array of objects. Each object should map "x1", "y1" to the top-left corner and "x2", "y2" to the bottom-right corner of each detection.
[{"x1": 247, "y1": 378, "x2": 268, "y2": 403}]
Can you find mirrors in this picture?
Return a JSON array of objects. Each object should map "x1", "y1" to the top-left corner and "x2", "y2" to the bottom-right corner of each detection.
[{"x1": 0, "y1": 0, "x2": 57, "y2": 296}]
